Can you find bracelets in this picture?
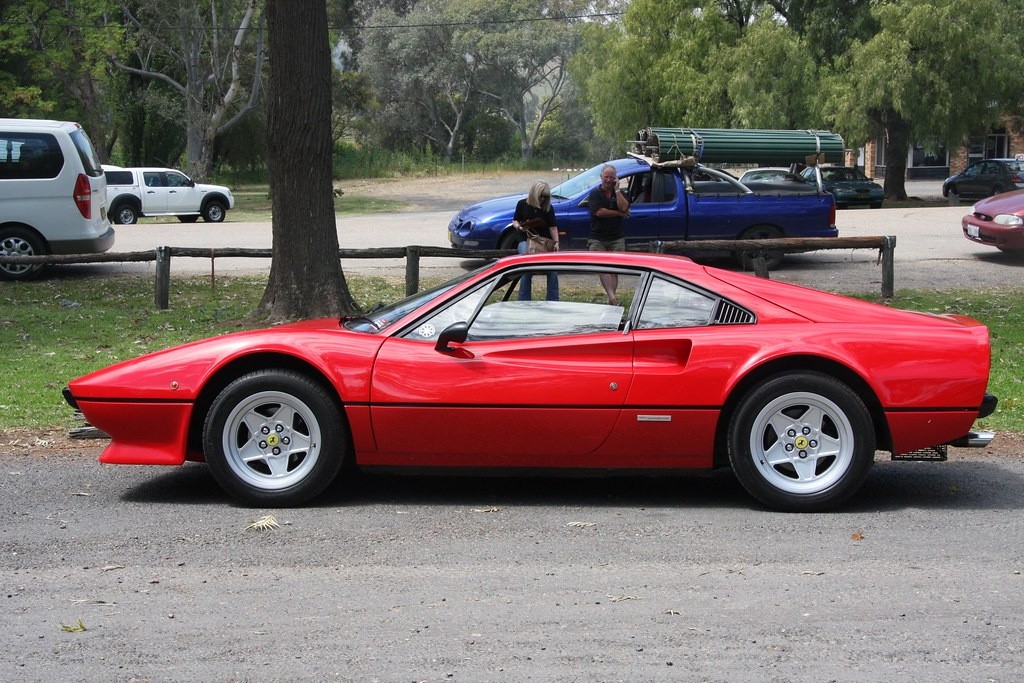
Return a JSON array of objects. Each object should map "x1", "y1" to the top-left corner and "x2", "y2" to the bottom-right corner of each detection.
[
  {"x1": 517, "y1": 226, "x2": 520, "y2": 230},
  {"x1": 615, "y1": 190, "x2": 620, "y2": 193}
]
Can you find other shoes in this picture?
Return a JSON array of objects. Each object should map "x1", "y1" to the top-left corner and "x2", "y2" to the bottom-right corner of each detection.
[{"x1": 609, "y1": 298, "x2": 622, "y2": 306}]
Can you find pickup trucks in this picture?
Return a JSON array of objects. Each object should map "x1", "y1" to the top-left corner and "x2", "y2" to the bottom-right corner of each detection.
[
  {"x1": 448, "y1": 158, "x2": 839, "y2": 268},
  {"x1": 102, "y1": 163, "x2": 234, "y2": 224}
]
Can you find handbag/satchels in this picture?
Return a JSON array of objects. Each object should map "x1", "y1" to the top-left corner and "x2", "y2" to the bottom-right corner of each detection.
[{"x1": 525, "y1": 227, "x2": 557, "y2": 253}]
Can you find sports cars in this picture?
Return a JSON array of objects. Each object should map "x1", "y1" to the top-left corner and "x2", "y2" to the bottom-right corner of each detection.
[{"x1": 62, "y1": 250, "x2": 999, "y2": 509}]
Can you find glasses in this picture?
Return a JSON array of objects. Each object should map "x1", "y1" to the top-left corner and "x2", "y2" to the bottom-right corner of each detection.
[{"x1": 540, "y1": 196, "x2": 549, "y2": 200}]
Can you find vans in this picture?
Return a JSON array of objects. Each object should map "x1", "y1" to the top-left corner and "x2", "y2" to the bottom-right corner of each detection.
[{"x1": 0, "y1": 116, "x2": 113, "y2": 276}]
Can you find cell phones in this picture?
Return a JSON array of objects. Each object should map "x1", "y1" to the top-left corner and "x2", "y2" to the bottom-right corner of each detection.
[{"x1": 612, "y1": 178, "x2": 617, "y2": 185}]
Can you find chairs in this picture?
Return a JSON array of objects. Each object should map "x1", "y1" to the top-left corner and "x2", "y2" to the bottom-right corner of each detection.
[{"x1": 633, "y1": 176, "x2": 652, "y2": 203}]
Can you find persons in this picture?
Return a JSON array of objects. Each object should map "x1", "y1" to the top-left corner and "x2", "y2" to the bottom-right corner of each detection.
[
  {"x1": 588, "y1": 165, "x2": 630, "y2": 306},
  {"x1": 513, "y1": 181, "x2": 560, "y2": 301}
]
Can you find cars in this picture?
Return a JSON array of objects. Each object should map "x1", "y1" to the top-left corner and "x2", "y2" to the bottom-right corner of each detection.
[
  {"x1": 961, "y1": 187, "x2": 1019, "y2": 248},
  {"x1": 737, "y1": 167, "x2": 810, "y2": 185},
  {"x1": 942, "y1": 158, "x2": 1024, "y2": 202},
  {"x1": 799, "y1": 165, "x2": 886, "y2": 209}
]
